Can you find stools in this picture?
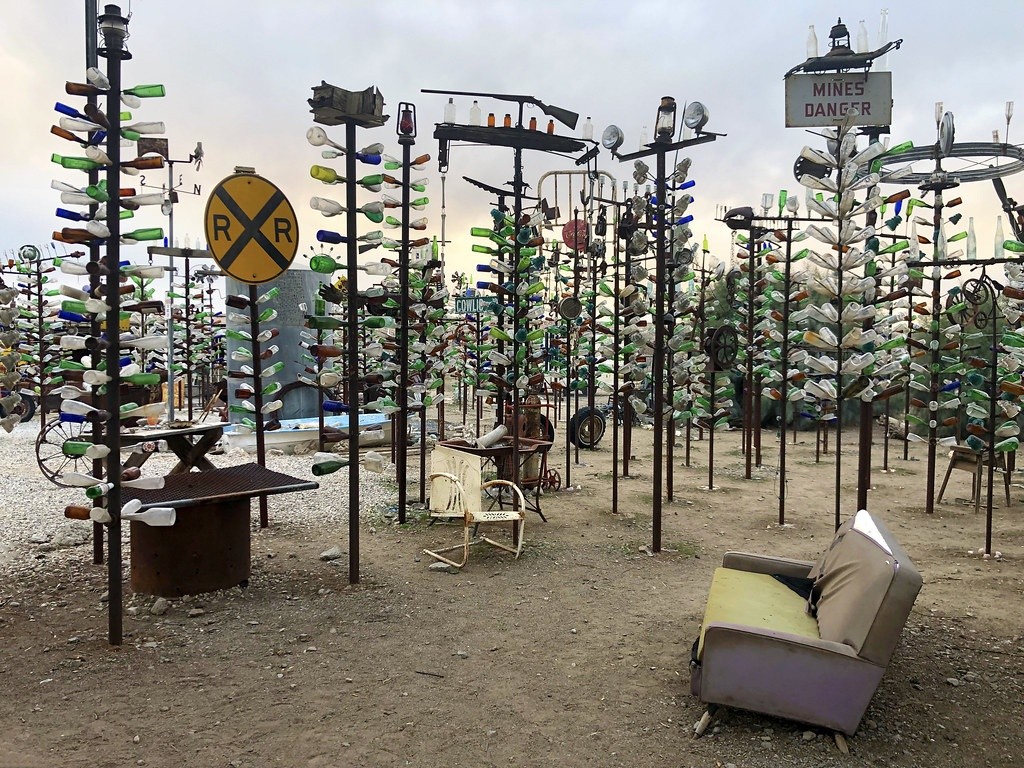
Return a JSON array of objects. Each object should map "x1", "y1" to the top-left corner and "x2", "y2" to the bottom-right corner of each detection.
[{"x1": 937, "y1": 444, "x2": 1012, "y2": 514}]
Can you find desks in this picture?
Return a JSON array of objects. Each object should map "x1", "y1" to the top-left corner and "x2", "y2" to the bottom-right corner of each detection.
[{"x1": 79, "y1": 421, "x2": 232, "y2": 476}]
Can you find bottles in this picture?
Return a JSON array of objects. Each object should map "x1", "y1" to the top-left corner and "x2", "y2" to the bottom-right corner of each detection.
[
  {"x1": 807, "y1": 25, "x2": 817, "y2": 59},
  {"x1": 994, "y1": 216, "x2": 1005, "y2": 258},
  {"x1": 8, "y1": 259, "x2": 14, "y2": 270},
  {"x1": 994, "y1": 262, "x2": 1024, "y2": 451},
  {"x1": 875, "y1": 9, "x2": 889, "y2": 51},
  {"x1": 1002, "y1": 240, "x2": 1024, "y2": 252},
  {"x1": 15, "y1": 68, "x2": 989, "y2": 525},
  {"x1": 992, "y1": 129, "x2": 1000, "y2": 143},
  {"x1": 857, "y1": 19, "x2": 869, "y2": 53},
  {"x1": 1005, "y1": 101, "x2": 1014, "y2": 125}
]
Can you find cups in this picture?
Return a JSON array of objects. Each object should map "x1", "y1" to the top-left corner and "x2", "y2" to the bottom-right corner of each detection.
[
  {"x1": 0, "y1": 372, "x2": 22, "y2": 390},
  {"x1": 0, "y1": 288, "x2": 20, "y2": 305},
  {"x1": 0, "y1": 394, "x2": 22, "y2": 414},
  {"x1": 0, "y1": 331, "x2": 22, "y2": 349},
  {"x1": 0, "y1": 353, "x2": 22, "y2": 371},
  {"x1": 0, "y1": 414, "x2": 22, "y2": 433},
  {"x1": 0, "y1": 309, "x2": 20, "y2": 325}
]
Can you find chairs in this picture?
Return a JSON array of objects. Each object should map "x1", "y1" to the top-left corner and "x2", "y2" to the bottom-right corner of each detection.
[
  {"x1": 608, "y1": 394, "x2": 614, "y2": 405},
  {"x1": 424, "y1": 445, "x2": 525, "y2": 568}
]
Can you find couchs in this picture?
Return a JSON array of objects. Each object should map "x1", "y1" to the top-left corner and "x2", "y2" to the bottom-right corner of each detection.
[{"x1": 688, "y1": 509, "x2": 924, "y2": 756}]
